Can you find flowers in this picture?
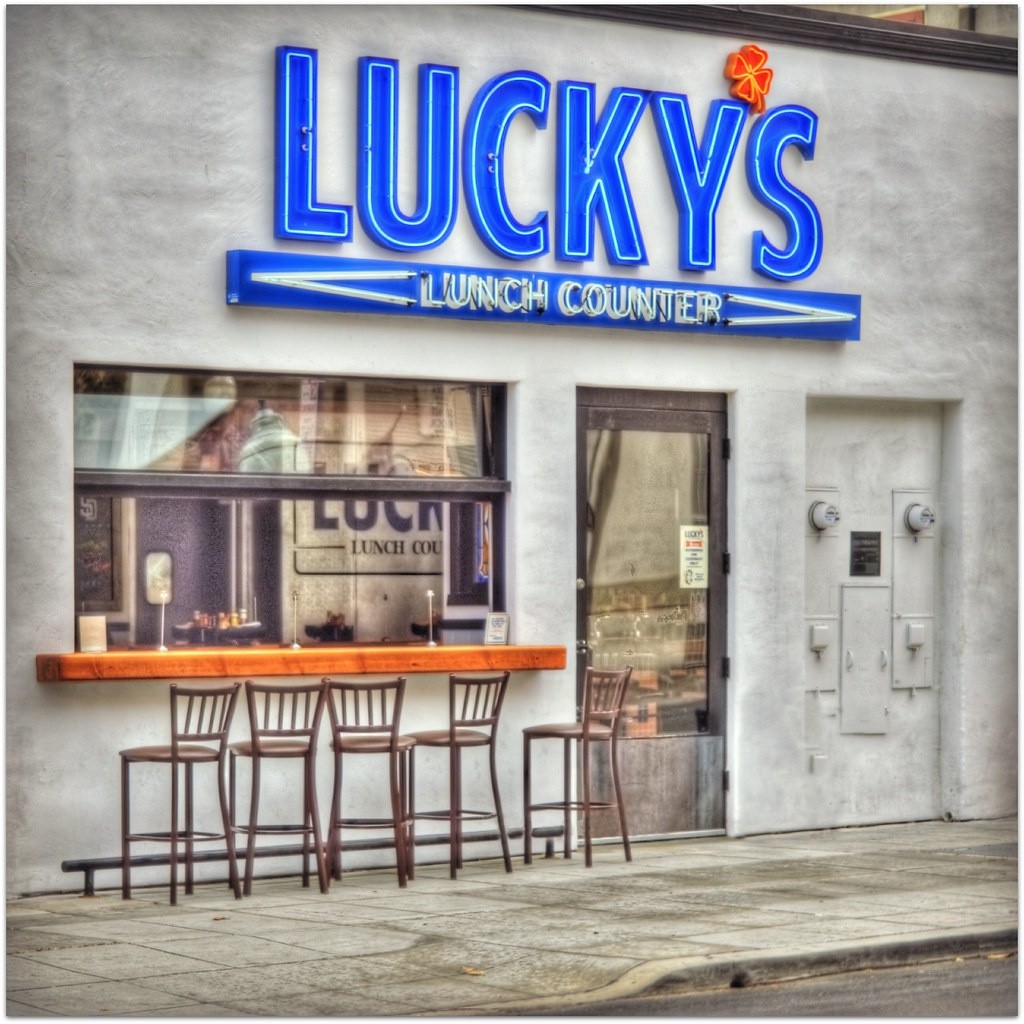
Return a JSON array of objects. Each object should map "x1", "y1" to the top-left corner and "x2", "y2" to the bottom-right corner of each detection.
[{"x1": 725, "y1": 44, "x2": 773, "y2": 112}]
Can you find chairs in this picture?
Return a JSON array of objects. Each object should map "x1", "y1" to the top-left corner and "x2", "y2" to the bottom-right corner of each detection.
[
  {"x1": 119, "y1": 682, "x2": 243, "y2": 906},
  {"x1": 522, "y1": 666, "x2": 635, "y2": 868},
  {"x1": 228, "y1": 677, "x2": 332, "y2": 897},
  {"x1": 321, "y1": 677, "x2": 415, "y2": 889},
  {"x1": 407, "y1": 670, "x2": 512, "y2": 877}
]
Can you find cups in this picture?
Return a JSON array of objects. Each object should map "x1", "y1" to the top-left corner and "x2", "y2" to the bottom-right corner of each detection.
[{"x1": 79, "y1": 616, "x2": 107, "y2": 653}]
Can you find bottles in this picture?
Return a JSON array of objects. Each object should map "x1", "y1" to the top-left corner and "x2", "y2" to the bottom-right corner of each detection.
[{"x1": 233, "y1": 407, "x2": 311, "y2": 474}]
